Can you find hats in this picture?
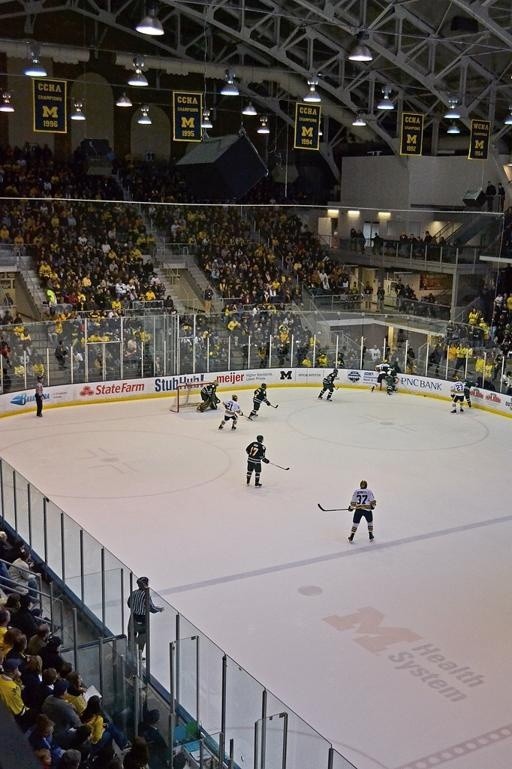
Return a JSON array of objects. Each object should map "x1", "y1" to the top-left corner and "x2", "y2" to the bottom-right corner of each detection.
[
  {"x1": 54, "y1": 679, "x2": 73, "y2": 692},
  {"x1": 3, "y1": 658, "x2": 22, "y2": 669},
  {"x1": 38, "y1": 375, "x2": 43, "y2": 379}
]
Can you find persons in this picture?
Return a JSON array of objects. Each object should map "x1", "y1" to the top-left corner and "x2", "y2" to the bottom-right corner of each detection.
[
  {"x1": 0, "y1": 525, "x2": 218, "y2": 768},
  {"x1": 243, "y1": 433, "x2": 272, "y2": 489},
  {"x1": 197, "y1": 379, "x2": 271, "y2": 431},
  {"x1": 318, "y1": 369, "x2": 339, "y2": 401},
  {"x1": 346, "y1": 478, "x2": 380, "y2": 545},
  {"x1": 450, "y1": 379, "x2": 472, "y2": 415},
  {"x1": 35, "y1": 375, "x2": 46, "y2": 417},
  {"x1": 0, "y1": 125, "x2": 512, "y2": 396}
]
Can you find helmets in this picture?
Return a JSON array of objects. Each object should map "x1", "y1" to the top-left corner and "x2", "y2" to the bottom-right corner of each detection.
[
  {"x1": 257, "y1": 435, "x2": 263, "y2": 442},
  {"x1": 137, "y1": 577, "x2": 148, "y2": 589},
  {"x1": 261, "y1": 383, "x2": 266, "y2": 389},
  {"x1": 360, "y1": 480, "x2": 367, "y2": 489},
  {"x1": 232, "y1": 394, "x2": 237, "y2": 400}
]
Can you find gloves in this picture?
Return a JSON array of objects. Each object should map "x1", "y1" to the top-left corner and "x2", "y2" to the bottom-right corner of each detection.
[
  {"x1": 266, "y1": 402, "x2": 271, "y2": 406},
  {"x1": 348, "y1": 506, "x2": 353, "y2": 511},
  {"x1": 263, "y1": 458, "x2": 269, "y2": 464}
]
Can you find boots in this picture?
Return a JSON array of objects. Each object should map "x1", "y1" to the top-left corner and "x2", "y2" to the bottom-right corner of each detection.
[
  {"x1": 369, "y1": 532, "x2": 374, "y2": 539},
  {"x1": 247, "y1": 476, "x2": 251, "y2": 484},
  {"x1": 348, "y1": 533, "x2": 354, "y2": 540},
  {"x1": 326, "y1": 392, "x2": 333, "y2": 402},
  {"x1": 255, "y1": 477, "x2": 262, "y2": 486},
  {"x1": 318, "y1": 389, "x2": 325, "y2": 399}
]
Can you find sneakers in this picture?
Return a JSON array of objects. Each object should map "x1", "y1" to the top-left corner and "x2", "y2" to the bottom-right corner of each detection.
[
  {"x1": 219, "y1": 426, "x2": 223, "y2": 428},
  {"x1": 450, "y1": 409, "x2": 456, "y2": 412},
  {"x1": 198, "y1": 407, "x2": 203, "y2": 412},
  {"x1": 232, "y1": 426, "x2": 236, "y2": 429},
  {"x1": 460, "y1": 408, "x2": 464, "y2": 412}
]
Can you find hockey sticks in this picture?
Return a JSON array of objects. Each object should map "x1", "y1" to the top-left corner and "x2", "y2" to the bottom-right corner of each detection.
[
  {"x1": 269, "y1": 462, "x2": 289, "y2": 471},
  {"x1": 318, "y1": 504, "x2": 349, "y2": 511},
  {"x1": 242, "y1": 413, "x2": 255, "y2": 422},
  {"x1": 271, "y1": 404, "x2": 278, "y2": 408}
]
